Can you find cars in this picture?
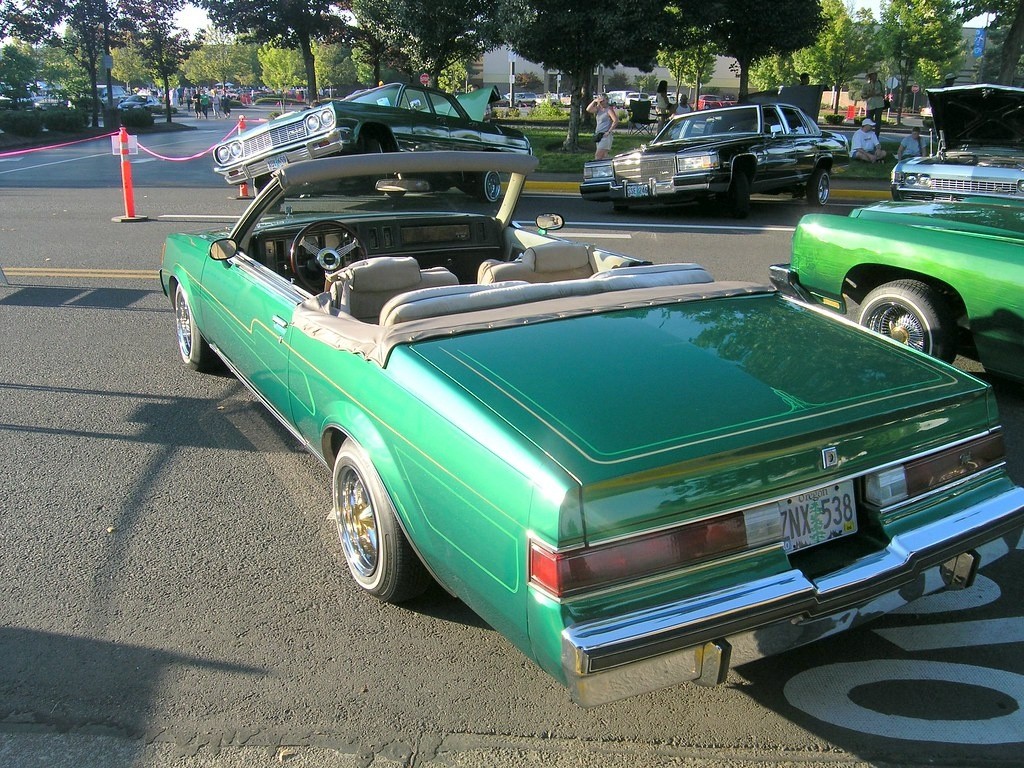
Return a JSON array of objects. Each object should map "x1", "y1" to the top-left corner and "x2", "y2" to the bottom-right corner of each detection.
[
  {"x1": 765, "y1": 192, "x2": 1022, "y2": 390},
  {"x1": 33, "y1": 93, "x2": 71, "y2": 111},
  {"x1": 919, "y1": 107, "x2": 933, "y2": 118},
  {"x1": 579, "y1": 82, "x2": 852, "y2": 217},
  {"x1": 890, "y1": 84, "x2": 1024, "y2": 200},
  {"x1": 118, "y1": 95, "x2": 163, "y2": 111},
  {"x1": 210, "y1": 80, "x2": 533, "y2": 213},
  {"x1": 499, "y1": 87, "x2": 740, "y2": 124}
]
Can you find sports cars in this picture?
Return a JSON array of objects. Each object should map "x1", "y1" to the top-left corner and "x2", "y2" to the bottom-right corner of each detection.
[{"x1": 155, "y1": 147, "x2": 1023, "y2": 713}]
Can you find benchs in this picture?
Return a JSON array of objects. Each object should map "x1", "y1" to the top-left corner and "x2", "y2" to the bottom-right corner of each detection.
[{"x1": 375, "y1": 262, "x2": 714, "y2": 326}]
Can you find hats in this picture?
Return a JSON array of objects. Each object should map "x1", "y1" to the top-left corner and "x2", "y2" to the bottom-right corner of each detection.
[
  {"x1": 865, "y1": 67, "x2": 880, "y2": 77},
  {"x1": 945, "y1": 74, "x2": 958, "y2": 80},
  {"x1": 862, "y1": 119, "x2": 876, "y2": 126}
]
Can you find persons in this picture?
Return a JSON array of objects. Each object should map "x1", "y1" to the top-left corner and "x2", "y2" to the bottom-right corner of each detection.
[
  {"x1": 655, "y1": 79, "x2": 671, "y2": 135},
  {"x1": 897, "y1": 126, "x2": 929, "y2": 162},
  {"x1": 469, "y1": 84, "x2": 494, "y2": 123},
  {"x1": 587, "y1": 93, "x2": 617, "y2": 159},
  {"x1": 673, "y1": 93, "x2": 695, "y2": 116},
  {"x1": 847, "y1": 117, "x2": 886, "y2": 165},
  {"x1": 798, "y1": 72, "x2": 810, "y2": 86},
  {"x1": 126, "y1": 82, "x2": 336, "y2": 120},
  {"x1": 860, "y1": 68, "x2": 887, "y2": 139}
]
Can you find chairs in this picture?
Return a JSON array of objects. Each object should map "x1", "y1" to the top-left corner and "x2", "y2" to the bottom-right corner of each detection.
[
  {"x1": 477, "y1": 241, "x2": 595, "y2": 286},
  {"x1": 330, "y1": 255, "x2": 461, "y2": 325},
  {"x1": 731, "y1": 119, "x2": 758, "y2": 132},
  {"x1": 627, "y1": 99, "x2": 659, "y2": 136}
]
